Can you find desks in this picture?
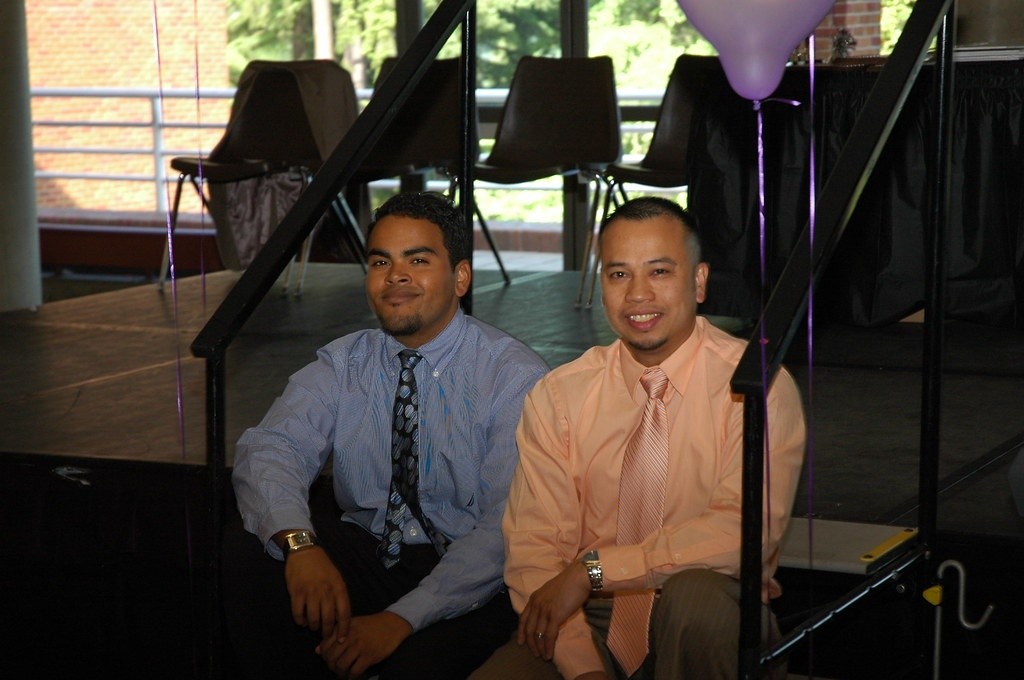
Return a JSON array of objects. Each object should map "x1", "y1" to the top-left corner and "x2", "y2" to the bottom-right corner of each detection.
[{"x1": 689, "y1": 59, "x2": 1024, "y2": 378}]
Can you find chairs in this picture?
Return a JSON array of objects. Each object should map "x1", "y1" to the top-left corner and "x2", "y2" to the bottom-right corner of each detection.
[
  {"x1": 367, "y1": 55, "x2": 512, "y2": 286},
  {"x1": 156, "y1": 59, "x2": 374, "y2": 298},
  {"x1": 585, "y1": 53, "x2": 719, "y2": 310},
  {"x1": 473, "y1": 55, "x2": 622, "y2": 309}
]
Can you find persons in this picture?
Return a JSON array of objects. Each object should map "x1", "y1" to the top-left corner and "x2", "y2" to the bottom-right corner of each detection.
[
  {"x1": 464, "y1": 196, "x2": 807, "y2": 680},
  {"x1": 224, "y1": 192, "x2": 553, "y2": 680}
]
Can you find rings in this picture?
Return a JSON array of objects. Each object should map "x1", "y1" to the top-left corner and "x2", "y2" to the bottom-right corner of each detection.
[{"x1": 533, "y1": 631, "x2": 546, "y2": 640}]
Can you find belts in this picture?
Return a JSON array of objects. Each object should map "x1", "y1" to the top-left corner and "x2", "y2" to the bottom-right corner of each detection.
[{"x1": 355, "y1": 526, "x2": 441, "y2": 569}]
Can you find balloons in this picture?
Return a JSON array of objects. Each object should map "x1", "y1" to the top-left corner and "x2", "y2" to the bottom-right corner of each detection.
[{"x1": 676, "y1": 0, "x2": 839, "y2": 110}]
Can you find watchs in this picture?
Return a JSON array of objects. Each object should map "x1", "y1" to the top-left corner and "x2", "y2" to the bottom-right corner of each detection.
[
  {"x1": 283, "y1": 529, "x2": 325, "y2": 559},
  {"x1": 581, "y1": 549, "x2": 605, "y2": 592}
]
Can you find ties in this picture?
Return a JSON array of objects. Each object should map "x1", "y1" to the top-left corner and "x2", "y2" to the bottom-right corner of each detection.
[
  {"x1": 604, "y1": 364, "x2": 670, "y2": 678},
  {"x1": 378, "y1": 348, "x2": 452, "y2": 571}
]
